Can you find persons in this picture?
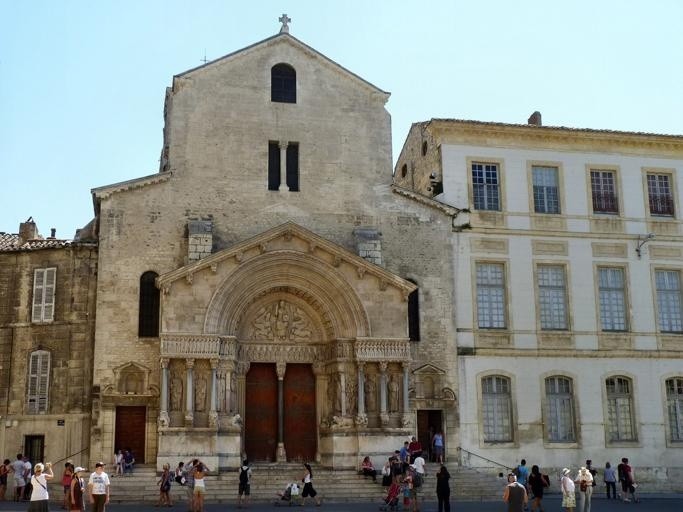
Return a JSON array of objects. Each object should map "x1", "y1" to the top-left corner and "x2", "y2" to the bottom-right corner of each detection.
[
  {"x1": 275, "y1": 301, "x2": 289, "y2": 339},
  {"x1": 381, "y1": 427, "x2": 450, "y2": 512},
  {"x1": 238, "y1": 459, "x2": 252, "y2": 507},
  {"x1": 123, "y1": 449, "x2": 135, "y2": 473},
  {"x1": 503, "y1": 458, "x2": 639, "y2": 512},
  {"x1": 362, "y1": 456, "x2": 377, "y2": 483},
  {"x1": 155, "y1": 459, "x2": 210, "y2": 512},
  {"x1": 388, "y1": 374, "x2": 399, "y2": 414},
  {"x1": 114, "y1": 450, "x2": 123, "y2": 475},
  {"x1": 0, "y1": 454, "x2": 86, "y2": 512},
  {"x1": 170, "y1": 373, "x2": 183, "y2": 412},
  {"x1": 300, "y1": 463, "x2": 321, "y2": 506},
  {"x1": 195, "y1": 372, "x2": 207, "y2": 412},
  {"x1": 88, "y1": 462, "x2": 110, "y2": 512}
]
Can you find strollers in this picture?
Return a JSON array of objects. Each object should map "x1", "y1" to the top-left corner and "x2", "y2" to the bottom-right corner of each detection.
[
  {"x1": 380, "y1": 482, "x2": 406, "y2": 511},
  {"x1": 274, "y1": 480, "x2": 300, "y2": 507}
]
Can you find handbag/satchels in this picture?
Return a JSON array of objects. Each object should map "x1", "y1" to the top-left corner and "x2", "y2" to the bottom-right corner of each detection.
[
  {"x1": 581, "y1": 484, "x2": 587, "y2": 491},
  {"x1": 512, "y1": 467, "x2": 520, "y2": 478}
]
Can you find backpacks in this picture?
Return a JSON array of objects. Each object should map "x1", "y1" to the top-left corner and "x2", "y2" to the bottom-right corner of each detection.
[
  {"x1": 238, "y1": 466, "x2": 250, "y2": 484},
  {"x1": 618, "y1": 464, "x2": 627, "y2": 481}
]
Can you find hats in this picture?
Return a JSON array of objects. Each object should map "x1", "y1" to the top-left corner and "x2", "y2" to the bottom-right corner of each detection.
[
  {"x1": 96, "y1": 462, "x2": 105, "y2": 467},
  {"x1": 563, "y1": 468, "x2": 570, "y2": 475},
  {"x1": 74, "y1": 466, "x2": 85, "y2": 473}
]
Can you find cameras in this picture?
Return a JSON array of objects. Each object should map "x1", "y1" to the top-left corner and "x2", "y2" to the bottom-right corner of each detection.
[{"x1": 46, "y1": 463, "x2": 51, "y2": 467}]
